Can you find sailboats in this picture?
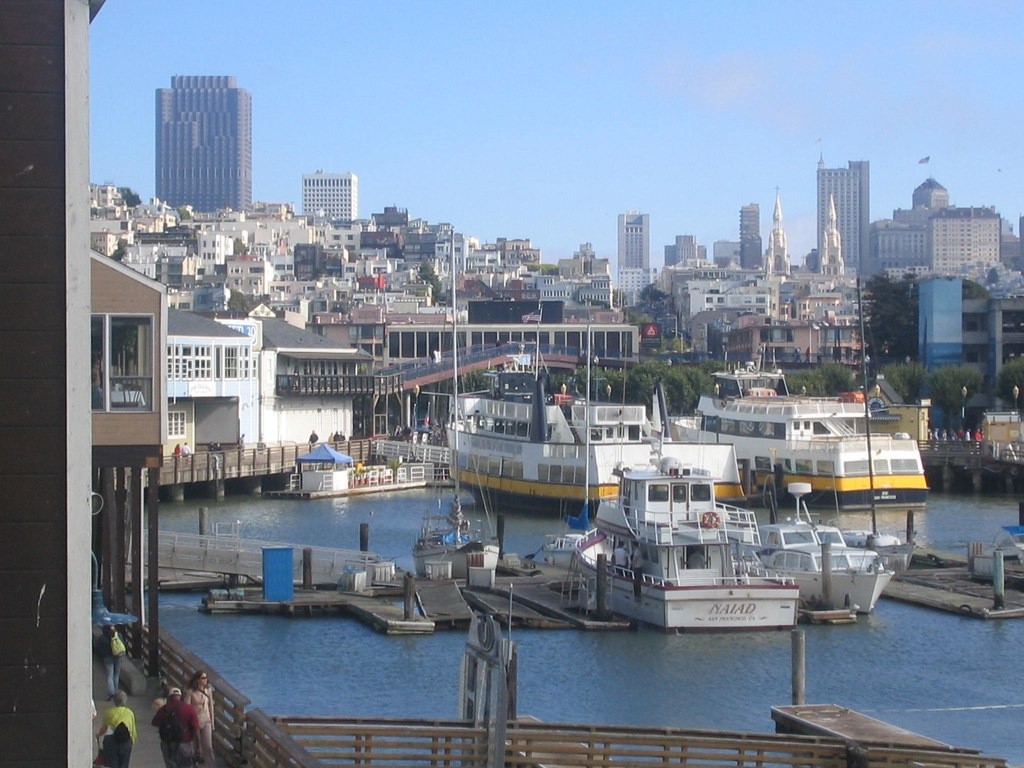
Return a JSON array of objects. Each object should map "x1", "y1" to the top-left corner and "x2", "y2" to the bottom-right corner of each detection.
[
  {"x1": 411, "y1": 226, "x2": 503, "y2": 579},
  {"x1": 525, "y1": 324, "x2": 595, "y2": 566}
]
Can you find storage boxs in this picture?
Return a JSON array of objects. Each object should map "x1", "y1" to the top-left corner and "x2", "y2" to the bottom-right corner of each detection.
[
  {"x1": 336, "y1": 570, "x2": 367, "y2": 592},
  {"x1": 503, "y1": 553, "x2": 520, "y2": 566},
  {"x1": 469, "y1": 567, "x2": 495, "y2": 588},
  {"x1": 374, "y1": 561, "x2": 396, "y2": 582},
  {"x1": 424, "y1": 560, "x2": 453, "y2": 581}
]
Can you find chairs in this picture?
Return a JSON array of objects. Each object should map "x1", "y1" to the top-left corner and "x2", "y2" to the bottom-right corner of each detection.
[
  {"x1": 383, "y1": 469, "x2": 393, "y2": 484},
  {"x1": 317, "y1": 474, "x2": 333, "y2": 491},
  {"x1": 369, "y1": 469, "x2": 378, "y2": 487},
  {"x1": 111, "y1": 383, "x2": 143, "y2": 407},
  {"x1": 285, "y1": 473, "x2": 301, "y2": 491},
  {"x1": 577, "y1": 576, "x2": 613, "y2": 617},
  {"x1": 411, "y1": 466, "x2": 425, "y2": 482},
  {"x1": 397, "y1": 468, "x2": 406, "y2": 483}
]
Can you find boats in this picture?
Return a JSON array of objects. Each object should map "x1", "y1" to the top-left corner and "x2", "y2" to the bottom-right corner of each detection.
[
  {"x1": 669, "y1": 341, "x2": 930, "y2": 514},
  {"x1": 570, "y1": 383, "x2": 798, "y2": 632},
  {"x1": 737, "y1": 480, "x2": 897, "y2": 615},
  {"x1": 446, "y1": 295, "x2": 747, "y2": 515}
]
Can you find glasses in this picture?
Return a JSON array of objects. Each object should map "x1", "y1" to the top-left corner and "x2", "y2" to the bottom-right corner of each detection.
[{"x1": 200, "y1": 676, "x2": 207, "y2": 679}]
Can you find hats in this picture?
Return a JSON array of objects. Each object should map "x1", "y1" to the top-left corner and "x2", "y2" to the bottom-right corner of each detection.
[
  {"x1": 168, "y1": 687, "x2": 182, "y2": 696},
  {"x1": 113, "y1": 690, "x2": 127, "y2": 705}
]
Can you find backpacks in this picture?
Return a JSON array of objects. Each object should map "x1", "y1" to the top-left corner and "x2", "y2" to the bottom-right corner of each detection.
[
  {"x1": 114, "y1": 721, "x2": 130, "y2": 742},
  {"x1": 159, "y1": 701, "x2": 189, "y2": 743},
  {"x1": 110, "y1": 631, "x2": 126, "y2": 656}
]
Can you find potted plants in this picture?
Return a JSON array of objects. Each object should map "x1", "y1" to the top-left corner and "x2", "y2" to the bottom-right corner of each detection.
[{"x1": 386, "y1": 457, "x2": 403, "y2": 481}]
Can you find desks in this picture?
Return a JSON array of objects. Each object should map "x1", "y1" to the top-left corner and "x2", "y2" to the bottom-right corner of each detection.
[{"x1": 111, "y1": 390, "x2": 146, "y2": 405}]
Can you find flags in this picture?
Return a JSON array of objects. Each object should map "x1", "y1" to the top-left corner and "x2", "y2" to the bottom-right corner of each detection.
[{"x1": 521, "y1": 308, "x2": 542, "y2": 324}]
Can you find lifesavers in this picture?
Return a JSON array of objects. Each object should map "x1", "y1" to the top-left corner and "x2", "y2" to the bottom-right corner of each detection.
[{"x1": 702, "y1": 512, "x2": 720, "y2": 528}]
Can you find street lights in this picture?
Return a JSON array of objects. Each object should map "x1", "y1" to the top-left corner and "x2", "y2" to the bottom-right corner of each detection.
[
  {"x1": 808, "y1": 321, "x2": 831, "y2": 363},
  {"x1": 1012, "y1": 385, "x2": 1020, "y2": 412},
  {"x1": 960, "y1": 386, "x2": 968, "y2": 430}
]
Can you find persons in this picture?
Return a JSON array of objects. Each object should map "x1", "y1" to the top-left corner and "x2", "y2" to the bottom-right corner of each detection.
[
  {"x1": 817, "y1": 348, "x2": 822, "y2": 362},
  {"x1": 92, "y1": 691, "x2": 137, "y2": 768},
  {"x1": 175, "y1": 443, "x2": 191, "y2": 465},
  {"x1": 239, "y1": 434, "x2": 245, "y2": 452},
  {"x1": 394, "y1": 424, "x2": 402, "y2": 436},
  {"x1": 688, "y1": 549, "x2": 705, "y2": 569},
  {"x1": 310, "y1": 430, "x2": 318, "y2": 446},
  {"x1": 611, "y1": 540, "x2": 644, "y2": 582},
  {"x1": 97, "y1": 624, "x2": 124, "y2": 701},
  {"x1": 356, "y1": 461, "x2": 363, "y2": 476},
  {"x1": 208, "y1": 441, "x2": 222, "y2": 451},
  {"x1": 928, "y1": 428, "x2": 985, "y2": 452},
  {"x1": 430, "y1": 347, "x2": 438, "y2": 364},
  {"x1": 833, "y1": 350, "x2": 842, "y2": 363},
  {"x1": 188, "y1": 670, "x2": 215, "y2": 768},
  {"x1": 151, "y1": 687, "x2": 203, "y2": 768},
  {"x1": 795, "y1": 347, "x2": 810, "y2": 362},
  {"x1": 329, "y1": 431, "x2": 345, "y2": 443},
  {"x1": 496, "y1": 341, "x2": 503, "y2": 356}
]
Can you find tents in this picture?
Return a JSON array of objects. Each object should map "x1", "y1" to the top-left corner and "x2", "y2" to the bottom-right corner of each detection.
[{"x1": 296, "y1": 443, "x2": 355, "y2": 487}]
